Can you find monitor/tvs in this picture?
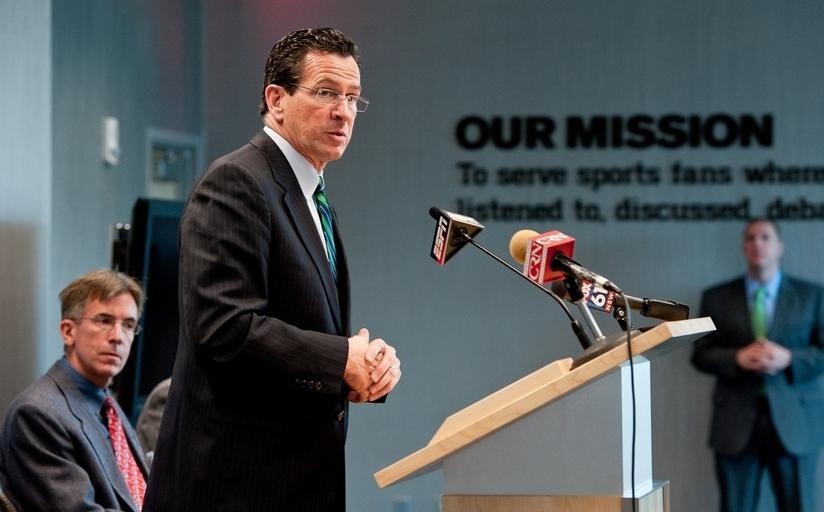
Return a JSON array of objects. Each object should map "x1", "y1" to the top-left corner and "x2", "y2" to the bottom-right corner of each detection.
[{"x1": 109, "y1": 195, "x2": 188, "y2": 429}]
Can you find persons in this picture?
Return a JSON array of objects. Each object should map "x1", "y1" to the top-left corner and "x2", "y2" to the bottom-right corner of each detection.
[
  {"x1": 688, "y1": 215, "x2": 823, "y2": 510},
  {"x1": 0, "y1": 265, "x2": 158, "y2": 511},
  {"x1": 140, "y1": 25, "x2": 402, "y2": 511},
  {"x1": 132, "y1": 373, "x2": 178, "y2": 453}
]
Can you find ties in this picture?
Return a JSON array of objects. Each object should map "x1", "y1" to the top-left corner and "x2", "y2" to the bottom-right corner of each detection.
[
  {"x1": 313, "y1": 175, "x2": 342, "y2": 313},
  {"x1": 751, "y1": 288, "x2": 768, "y2": 339},
  {"x1": 100, "y1": 396, "x2": 148, "y2": 512}
]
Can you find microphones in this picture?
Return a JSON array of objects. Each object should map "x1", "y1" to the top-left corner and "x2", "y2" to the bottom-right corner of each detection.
[
  {"x1": 505, "y1": 220, "x2": 616, "y2": 294},
  {"x1": 548, "y1": 272, "x2": 646, "y2": 315},
  {"x1": 423, "y1": 203, "x2": 593, "y2": 348}
]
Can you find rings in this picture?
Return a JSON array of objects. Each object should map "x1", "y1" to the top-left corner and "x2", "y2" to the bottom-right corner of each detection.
[{"x1": 391, "y1": 365, "x2": 399, "y2": 370}]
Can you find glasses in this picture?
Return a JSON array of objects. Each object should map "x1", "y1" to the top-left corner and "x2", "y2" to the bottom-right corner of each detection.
[
  {"x1": 295, "y1": 84, "x2": 370, "y2": 113},
  {"x1": 81, "y1": 315, "x2": 141, "y2": 336}
]
